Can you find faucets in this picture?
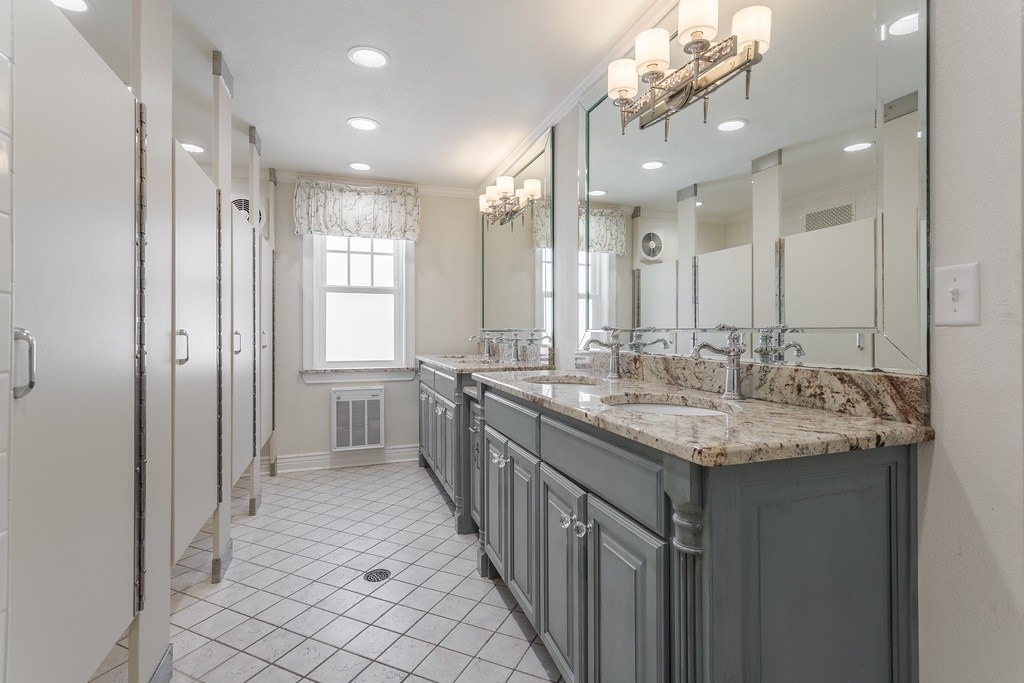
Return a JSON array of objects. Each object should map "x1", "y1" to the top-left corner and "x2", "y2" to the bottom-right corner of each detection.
[
  {"x1": 690, "y1": 323, "x2": 746, "y2": 399},
  {"x1": 525, "y1": 328, "x2": 551, "y2": 345},
  {"x1": 493, "y1": 332, "x2": 511, "y2": 342},
  {"x1": 583, "y1": 325, "x2": 624, "y2": 379},
  {"x1": 498, "y1": 328, "x2": 521, "y2": 361},
  {"x1": 752, "y1": 323, "x2": 805, "y2": 363},
  {"x1": 628, "y1": 325, "x2": 670, "y2": 356},
  {"x1": 468, "y1": 328, "x2": 493, "y2": 357}
]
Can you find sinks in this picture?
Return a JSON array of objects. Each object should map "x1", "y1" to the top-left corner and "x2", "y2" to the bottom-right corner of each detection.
[
  {"x1": 600, "y1": 394, "x2": 742, "y2": 415},
  {"x1": 434, "y1": 355, "x2": 483, "y2": 359},
  {"x1": 454, "y1": 360, "x2": 506, "y2": 364},
  {"x1": 521, "y1": 376, "x2": 608, "y2": 385}
]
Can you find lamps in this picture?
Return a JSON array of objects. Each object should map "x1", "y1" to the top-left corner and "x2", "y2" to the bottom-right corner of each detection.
[
  {"x1": 500, "y1": 179, "x2": 541, "y2": 232},
  {"x1": 638, "y1": 6, "x2": 772, "y2": 142},
  {"x1": 607, "y1": 0, "x2": 736, "y2": 136},
  {"x1": 479, "y1": 175, "x2": 514, "y2": 231}
]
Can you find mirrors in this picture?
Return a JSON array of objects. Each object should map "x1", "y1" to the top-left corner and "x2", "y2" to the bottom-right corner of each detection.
[
  {"x1": 576, "y1": 0, "x2": 928, "y2": 379},
  {"x1": 474, "y1": 126, "x2": 553, "y2": 349}
]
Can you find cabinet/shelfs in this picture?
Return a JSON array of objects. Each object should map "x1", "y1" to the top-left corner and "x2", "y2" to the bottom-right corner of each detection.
[
  {"x1": 418, "y1": 360, "x2": 468, "y2": 534},
  {"x1": 539, "y1": 410, "x2": 917, "y2": 682},
  {"x1": 467, "y1": 382, "x2": 538, "y2": 637}
]
